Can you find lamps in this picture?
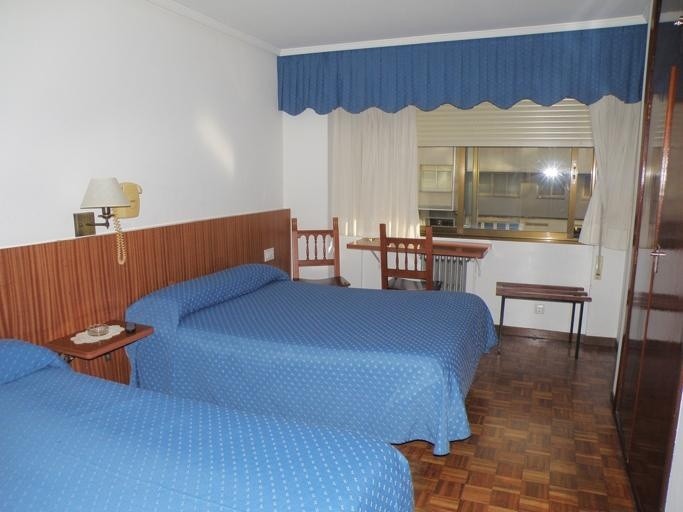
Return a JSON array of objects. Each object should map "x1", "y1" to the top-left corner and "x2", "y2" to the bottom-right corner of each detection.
[{"x1": 72, "y1": 176, "x2": 131, "y2": 237}]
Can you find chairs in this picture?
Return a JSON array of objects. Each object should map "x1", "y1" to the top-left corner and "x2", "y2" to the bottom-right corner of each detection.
[
  {"x1": 288, "y1": 216, "x2": 352, "y2": 289},
  {"x1": 375, "y1": 223, "x2": 443, "y2": 291}
]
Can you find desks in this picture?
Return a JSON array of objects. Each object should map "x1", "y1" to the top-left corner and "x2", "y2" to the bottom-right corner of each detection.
[
  {"x1": 347, "y1": 238, "x2": 491, "y2": 271},
  {"x1": 44, "y1": 316, "x2": 153, "y2": 362}
]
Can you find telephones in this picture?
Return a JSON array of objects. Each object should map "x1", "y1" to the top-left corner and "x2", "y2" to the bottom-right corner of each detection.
[{"x1": 113, "y1": 182, "x2": 142, "y2": 219}]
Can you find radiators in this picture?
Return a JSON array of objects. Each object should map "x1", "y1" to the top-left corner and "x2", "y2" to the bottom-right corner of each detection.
[{"x1": 420, "y1": 252, "x2": 467, "y2": 292}]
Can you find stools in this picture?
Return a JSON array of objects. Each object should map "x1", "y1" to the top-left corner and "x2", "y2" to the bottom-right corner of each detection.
[{"x1": 494, "y1": 282, "x2": 591, "y2": 359}]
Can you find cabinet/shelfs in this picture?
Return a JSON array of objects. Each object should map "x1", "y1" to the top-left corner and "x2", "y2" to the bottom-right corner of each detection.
[{"x1": 611, "y1": 3, "x2": 683, "y2": 510}]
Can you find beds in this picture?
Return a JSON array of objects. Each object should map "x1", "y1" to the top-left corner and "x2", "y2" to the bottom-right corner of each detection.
[
  {"x1": 0, "y1": 336, "x2": 415, "y2": 512},
  {"x1": 120, "y1": 261, "x2": 499, "y2": 458}
]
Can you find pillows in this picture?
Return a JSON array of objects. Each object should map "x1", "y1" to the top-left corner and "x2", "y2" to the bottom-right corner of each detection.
[
  {"x1": 124, "y1": 263, "x2": 289, "y2": 334},
  {"x1": 0, "y1": 339, "x2": 72, "y2": 387}
]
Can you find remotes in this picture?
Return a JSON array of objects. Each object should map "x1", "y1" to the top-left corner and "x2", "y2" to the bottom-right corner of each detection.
[{"x1": 125, "y1": 322, "x2": 136, "y2": 333}]
